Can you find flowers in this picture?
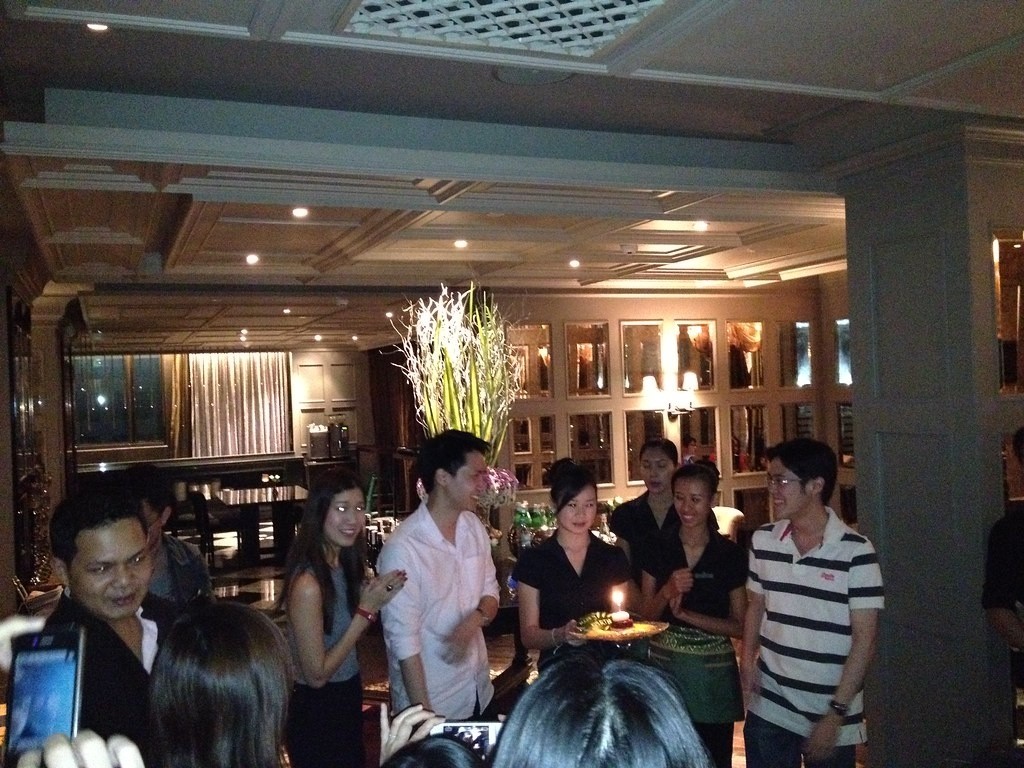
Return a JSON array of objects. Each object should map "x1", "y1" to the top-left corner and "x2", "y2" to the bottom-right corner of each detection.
[{"x1": 383, "y1": 281, "x2": 531, "y2": 507}]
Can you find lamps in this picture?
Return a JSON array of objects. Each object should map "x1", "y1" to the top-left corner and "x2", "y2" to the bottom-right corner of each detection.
[{"x1": 641, "y1": 372, "x2": 699, "y2": 419}]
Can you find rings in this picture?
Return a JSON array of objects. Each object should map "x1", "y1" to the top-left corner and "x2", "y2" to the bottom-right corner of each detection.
[
  {"x1": 389, "y1": 734, "x2": 396, "y2": 737},
  {"x1": 388, "y1": 585, "x2": 393, "y2": 590}
]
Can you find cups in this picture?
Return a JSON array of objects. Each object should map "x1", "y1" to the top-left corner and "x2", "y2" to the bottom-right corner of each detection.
[{"x1": 309, "y1": 430, "x2": 330, "y2": 459}]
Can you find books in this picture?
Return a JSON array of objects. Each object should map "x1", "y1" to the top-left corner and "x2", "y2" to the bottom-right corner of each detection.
[{"x1": 171, "y1": 479, "x2": 294, "y2": 506}]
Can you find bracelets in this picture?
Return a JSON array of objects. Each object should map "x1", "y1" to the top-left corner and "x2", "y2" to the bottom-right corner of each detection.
[
  {"x1": 475, "y1": 608, "x2": 491, "y2": 627},
  {"x1": 354, "y1": 608, "x2": 376, "y2": 622},
  {"x1": 831, "y1": 701, "x2": 849, "y2": 715},
  {"x1": 552, "y1": 628, "x2": 562, "y2": 653}
]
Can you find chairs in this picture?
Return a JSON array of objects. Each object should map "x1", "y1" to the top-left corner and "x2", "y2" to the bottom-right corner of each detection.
[{"x1": 152, "y1": 481, "x2": 215, "y2": 559}]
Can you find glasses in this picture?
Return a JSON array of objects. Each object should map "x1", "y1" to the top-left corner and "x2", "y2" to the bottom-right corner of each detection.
[{"x1": 767, "y1": 475, "x2": 805, "y2": 488}]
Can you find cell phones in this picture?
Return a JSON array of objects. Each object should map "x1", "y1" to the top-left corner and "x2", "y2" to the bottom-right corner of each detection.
[{"x1": 5, "y1": 623, "x2": 85, "y2": 768}]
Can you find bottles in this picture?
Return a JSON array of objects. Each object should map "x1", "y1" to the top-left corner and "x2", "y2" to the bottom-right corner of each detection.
[
  {"x1": 597, "y1": 496, "x2": 623, "y2": 537},
  {"x1": 328, "y1": 416, "x2": 350, "y2": 460},
  {"x1": 512, "y1": 499, "x2": 557, "y2": 527},
  {"x1": 518, "y1": 528, "x2": 530, "y2": 546}
]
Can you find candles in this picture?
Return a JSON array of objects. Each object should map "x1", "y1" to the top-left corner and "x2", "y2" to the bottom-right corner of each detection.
[{"x1": 611, "y1": 590, "x2": 629, "y2": 622}]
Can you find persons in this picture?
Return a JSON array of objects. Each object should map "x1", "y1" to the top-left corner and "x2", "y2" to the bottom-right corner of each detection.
[
  {"x1": 640, "y1": 459, "x2": 748, "y2": 768},
  {"x1": 454, "y1": 726, "x2": 488, "y2": 760},
  {"x1": 682, "y1": 434, "x2": 701, "y2": 464},
  {"x1": 516, "y1": 458, "x2": 638, "y2": 678},
  {"x1": 274, "y1": 470, "x2": 408, "y2": 768},
  {"x1": 489, "y1": 654, "x2": 716, "y2": 768},
  {"x1": 609, "y1": 438, "x2": 678, "y2": 563},
  {"x1": 983, "y1": 428, "x2": 1024, "y2": 649},
  {"x1": 152, "y1": 603, "x2": 290, "y2": 768},
  {"x1": 741, "y1": 437, "x2": 885, "y2": 768},
  {"x1": 375, "y1": 428, "x2": 500, "y2": 739},
  {"x1": 379, "y1": 703, "x2": 508, "y2": 768},
  {"x1": 0, "y1": 459, "x2": 214, "y2": 768}
]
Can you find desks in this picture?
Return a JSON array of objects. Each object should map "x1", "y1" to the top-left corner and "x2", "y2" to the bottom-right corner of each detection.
[
  {"x1": 476, "y1": 580, "x2": 526, "y2": 690},
  {"x1": 304, "y1": 460, "x2": 357, "y2": 492},
  {"x1": 215, "y1": 486, "x2": 306, "y2": 565}
]
